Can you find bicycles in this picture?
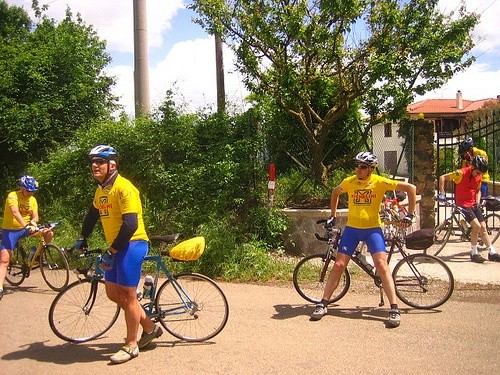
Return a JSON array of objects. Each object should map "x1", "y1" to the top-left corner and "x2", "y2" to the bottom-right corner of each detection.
[
  {"x1": 378, "y1": 193, "x2": 409, "y2": 254},
  {"x1": 424, "y1": 193, "x2": 500, "y2": 256},
  {"x1": 294, "y1": 217, "x2": 456, "y2": 310},
  {"x1": 48, "y1": 233, "x2": 229, "y2": 343},
  {"x1": 0, "y1": 229, "x2": 70, "y2": 292}
]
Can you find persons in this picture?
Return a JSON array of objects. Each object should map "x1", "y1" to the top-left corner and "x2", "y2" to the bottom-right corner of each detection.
[
  {"x1": 75, "y1": 146, "x2": 163, "y2": 362},
  {"x1": 459, "y1": 138, "x2": 489, "y2": 196},
  {"x1": 0, "y1": 176, "x2": 53, "y2": 298},
  {"x1": 311, "y1": 152, "x2": 417, "y2": 326},
  {"x1": 439, "y1": 156, "x2": 500, "y2": 262}
]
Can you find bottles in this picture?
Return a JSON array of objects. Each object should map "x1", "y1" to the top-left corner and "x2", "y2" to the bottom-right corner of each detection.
[
  {"x1": 143, "y1": 276, "x2": 154, "y2": 299},
  {"x1": 461, "y1": 220, "x2": 468, "y2": 232},
  {"x1": 136, "y1": 289, "x2": 143, "y2": 300},
  {"x1": 354, "y1": 250, "x2": 374, "y2": 271},
  {"x1": 28, "y1": 246, "x2": 37, "y2": 262}
]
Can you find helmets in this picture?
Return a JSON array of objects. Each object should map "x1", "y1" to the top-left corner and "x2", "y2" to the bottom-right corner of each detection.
[
  {"x1": 472, "y1": 155, "x2": 488, "y2": 173},
  {"x1": 458, "y1": 137, "x2": 473, "y2": 155},
  {"x1": 18, "y1": 175, "x2": 39, "y2": 192},
  {"x1": 352, "y1": 152, "x2": 380, "y2": 167},
  {"x1": 88, "y1": 145, "x2": 120, "y2": 159}
]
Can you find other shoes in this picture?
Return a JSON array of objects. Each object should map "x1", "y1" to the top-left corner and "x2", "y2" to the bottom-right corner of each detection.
[
  {"x1": 470, "y1": 253, "x2": 486, "y2": 263},
  {"x1": 488, "y1": 253, "x2": 500, "y2": 262}
]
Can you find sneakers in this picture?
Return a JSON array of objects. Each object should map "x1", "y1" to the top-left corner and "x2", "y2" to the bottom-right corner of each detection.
[
  {"x1": 109, "y1": 344, "x2": 139, "y2": 363},
  {"x1": 32, "y1": 254, "x2": 49, "y2": 265},
  {"x1": 310, "y1": 303, "x2": 327, "y2": 321},
  {"x1": 138, "y1": 325, "x2": 163, "y2": 349},
  {"x1": 0, "y1": 287, "x2": 4, "y2": 300},
  {"x1": 387, "y1": 309, "x2": 401, "y2": 328}
]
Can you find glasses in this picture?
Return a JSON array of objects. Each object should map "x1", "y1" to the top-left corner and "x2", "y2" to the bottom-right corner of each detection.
[
  {"x1": 92, "y1": 158, "x2": 108, "y2": 165},
  {"x1": 354, "y1": 163, "x2": 368, "y2": 169},
  {"x1": 475, "y1": 171, "x2": 482, "y2": 175}
]
